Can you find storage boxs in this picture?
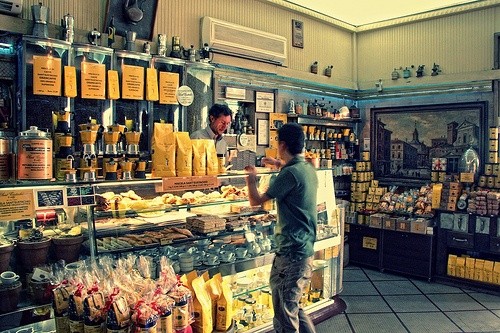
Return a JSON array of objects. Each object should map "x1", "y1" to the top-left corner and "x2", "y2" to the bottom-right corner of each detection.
[
  {"x1": 366, "y1": 180, "x2": 387, "y2": 210},
  {"x1": 447, "y1": 182, "x2": 463, "y2": 211},
  {"x1": 370, "y1": 214, "x2": 432, "y2": 234},
  {"x1": 447, "y1": 254, "x2": 500, "y2": 285}
]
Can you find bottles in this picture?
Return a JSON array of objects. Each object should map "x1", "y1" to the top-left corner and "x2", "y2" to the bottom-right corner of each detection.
[
  {"x1": 91, "y1": 28, "x2": 101, "y2": 46},
  {"x1": 188, "y1": 45, "x2": 195, "y2": 62},
  {"x1": 144, "y1": 42, "x2": 150, "y2": 55},
  {"x1": 290, "y1": 97, "x2": 340, "y2": 119},
  {"x1": 0, "y1": 125, "x2": 53, "y2": 182},
  {"x1": 311, "y1": 63, "x2": 317, "y2": 74},
  {"x1": 325, "y1": 66, "x2": 331, "y2": 77},
  {"x1": 107, "y1": 16, "x2": 116, "y2": 48},
  {"x1": 53, "y1": 110, "x2": 141, "y2": 180},
  {"x1": 392, "y1": 67, "x2": 411, "y2": 79}
]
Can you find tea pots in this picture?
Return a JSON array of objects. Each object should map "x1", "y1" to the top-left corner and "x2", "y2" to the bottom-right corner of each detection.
[
  {"x1": 246, "y1": 241, "x2": 262, "y2": 256},
  {"x1": 259, "y1": 234, "x2": 272, "y2": 252},
  {"x1": 124, "y1": 28, "x2": 136, "y2": 42}
]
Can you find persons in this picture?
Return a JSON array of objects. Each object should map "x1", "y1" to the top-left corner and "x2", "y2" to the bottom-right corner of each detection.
[
  {"x1": 245, "y1": 123, "x2": 318, "y2": 333},
  {"x1": 190, "y1": 103, "x2": 232, "y2": 164}
]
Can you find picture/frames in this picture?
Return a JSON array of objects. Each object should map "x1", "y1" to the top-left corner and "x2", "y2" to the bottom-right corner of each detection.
[{"x1": 370, "y1": 101, "x2": 488, "y2": 187}]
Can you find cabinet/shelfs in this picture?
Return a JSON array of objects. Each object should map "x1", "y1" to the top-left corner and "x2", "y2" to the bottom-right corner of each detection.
[
  {"x1": 0, "y1": 168, "x2": 347, "y2": 333},
  {"x1": 432, "y1": 207, "x2": 500, "y2": 296},
  {"x1": 286, "y1": 114, "x2": 362, "y2": 201}
]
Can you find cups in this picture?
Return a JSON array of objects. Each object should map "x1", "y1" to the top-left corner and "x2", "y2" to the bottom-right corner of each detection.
[
  {"x1": 158, "y1": 34, "x2": 167, "y2": 57},
  {"x1": 65, "y1": 262, "x2": 83, "y2": 273},
  {"x1": 1, "y1": 271, "x2": 20, "y2": 284},
  {"x1": 62, "y1": 12, "x2": 74, "y2": 44},
  {"x1": 110, "y1": 239, "x2": 248, "y2": 271}
]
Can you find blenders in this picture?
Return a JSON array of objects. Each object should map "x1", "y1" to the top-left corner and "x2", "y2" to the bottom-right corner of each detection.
[{"x1": 32, "y1": 3, "x2": 49, "y2": 37}]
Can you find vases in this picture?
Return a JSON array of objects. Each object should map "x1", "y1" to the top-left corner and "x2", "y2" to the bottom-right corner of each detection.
[
  {"x1": 52, "y1": 230, "x2": 84, "y2": 265},
  {"x1": 0, "y1": 281, "x2": 22, "y2": 314},
  {"x1": 18, "y1": 236, "x2": 52, "y2": 267},
  {"x1": 0, "y1": 240, "x2": 14, "y2": 273}
]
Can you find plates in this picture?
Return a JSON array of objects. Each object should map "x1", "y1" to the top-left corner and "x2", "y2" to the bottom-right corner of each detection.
[
  {"x1": 203, "y1": 261, "x2": 221, "y2": 266},
  {"x1": 234, "y1": 253, "x2": 252, "y2": 259},
  {"x1": 219, "y1": 257, "x2": 235, "y2": 262}
]
